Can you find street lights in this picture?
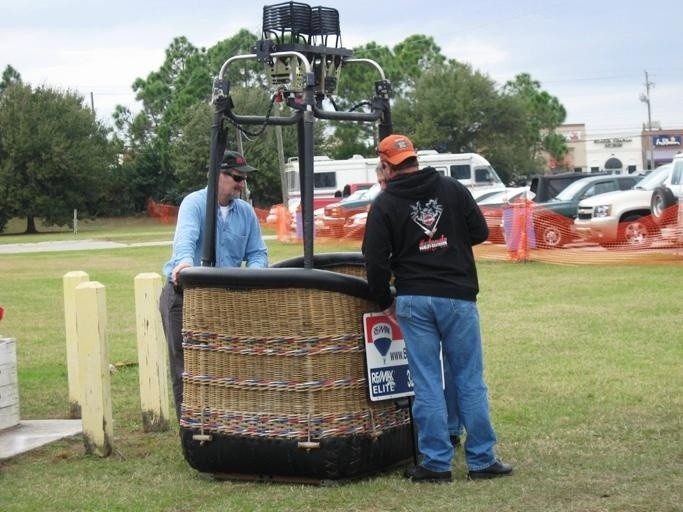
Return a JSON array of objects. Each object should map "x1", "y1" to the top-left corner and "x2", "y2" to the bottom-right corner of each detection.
[{"x1": 638, "y1": 94, "x2": 657, "y2": 172}]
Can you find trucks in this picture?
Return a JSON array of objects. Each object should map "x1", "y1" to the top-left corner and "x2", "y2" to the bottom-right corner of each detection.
[{"x1": 282, "y1": 144, "x2": 506, "y2": 233}]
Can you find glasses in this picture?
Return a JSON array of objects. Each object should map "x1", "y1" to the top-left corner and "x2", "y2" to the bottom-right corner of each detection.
[{"x1": 224, "y1": 171, "x2": 247, "y2": 181}]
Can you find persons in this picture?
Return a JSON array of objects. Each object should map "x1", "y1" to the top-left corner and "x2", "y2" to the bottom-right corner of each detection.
[
  {"x1": 376, "y1": 162, "x2": 463, "y2": 447},
  {"x1": 159, "y1": 151, "x2": 268, "y2": 428},
  {"x1": 361, "y1": 133, "x2": 513, "y2": 483}
]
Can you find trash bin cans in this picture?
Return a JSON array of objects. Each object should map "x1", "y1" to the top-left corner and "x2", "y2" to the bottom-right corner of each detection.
[
  {"x1": 296, "y1": 211, "x2": 316, "y2": 240},
  {"x1": 500, "y1": 204, "x2": 537, "y2": 251}
]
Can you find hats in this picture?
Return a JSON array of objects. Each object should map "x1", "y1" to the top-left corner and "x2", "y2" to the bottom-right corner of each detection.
[
  {"x1": 377, "y1": 134, "x2": 417, "y2": 165},
  {"x1": 219, "y1": 151, "x2": 258, "y2": 174}
]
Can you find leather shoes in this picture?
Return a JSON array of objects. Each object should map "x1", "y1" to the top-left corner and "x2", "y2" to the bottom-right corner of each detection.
[
  {"x1": 450, "y1": 435, "x2": 461, "y2": 447},
  {"x1": 404, "y1": 465, "x2": 451, "y2": 480},
  {"x1": 470, "y1": 462, "x2": 512, "y2": 479}
]
